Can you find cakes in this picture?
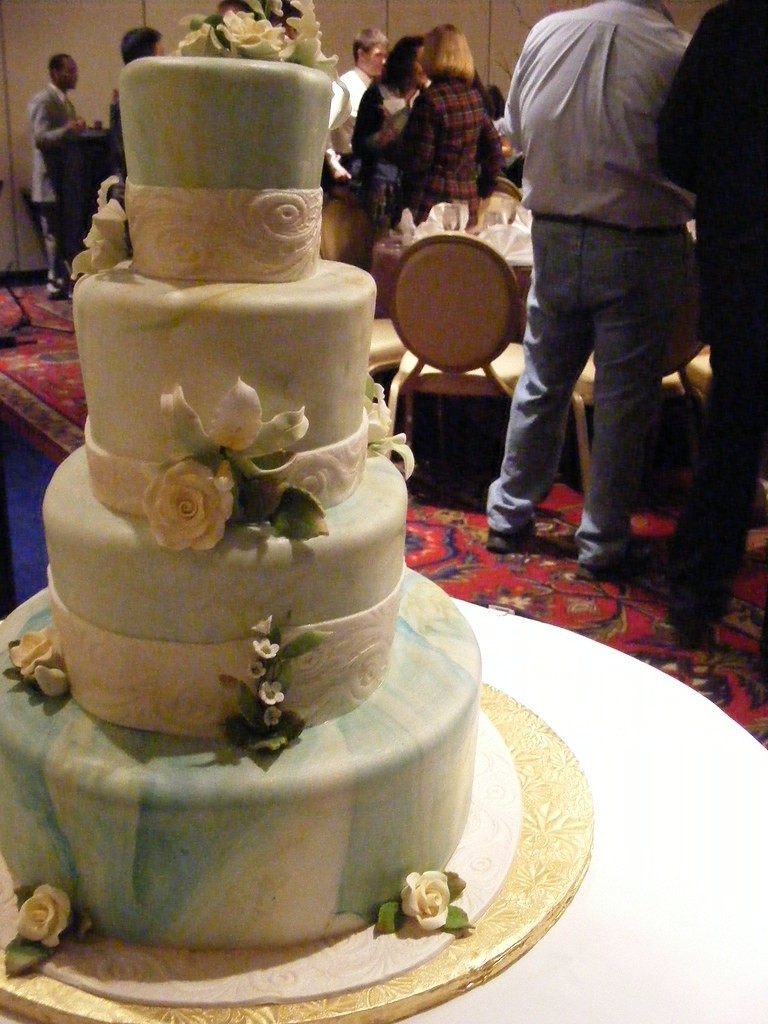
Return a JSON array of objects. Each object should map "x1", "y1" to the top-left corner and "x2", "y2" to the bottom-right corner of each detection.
[{"x1": 0, "y1": 0, "x2": 485, "y2": 970}]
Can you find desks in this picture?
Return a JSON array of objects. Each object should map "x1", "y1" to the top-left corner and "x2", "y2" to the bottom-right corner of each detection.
[
  {"x1": 0, "y1": 597, "x2": 767, "y2": 1023},
  {"x1": 370, "y1": 226, "x2": 535, "y2": 344}
]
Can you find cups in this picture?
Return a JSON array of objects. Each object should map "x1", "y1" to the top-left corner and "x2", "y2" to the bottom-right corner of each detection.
[
  {"x1": 443, "y1": 204, "x2": 463, "y2": 232},
  {"x1": 481, "y1": 209, "x2": 506, "y2": 234}
]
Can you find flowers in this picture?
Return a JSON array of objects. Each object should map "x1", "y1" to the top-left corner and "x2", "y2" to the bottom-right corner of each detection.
[
  {"x1": 176, "y1": 0, "x2": 352, "y2": 132},
  {"x1": 70, "y1": 176, "x2": 133, "y2": 280},
  {"x1": 363, "y1": 367, "x2": 415, "y2": 481},
  {"x1": 215, "y1": 614, "x2": 337, "y2": 754},
  {"x1": 142, "y1": 376, "x2": 329, "y2": 553},
  {"x1": 372, "y1": 870, "x2": 476, "y2": 939},
  {"x1": 8, "y1": 624, "x2": 67, "y2": 698},
  {"x1": 4, "y1": 882, "x2": 93, "y2": 978}
]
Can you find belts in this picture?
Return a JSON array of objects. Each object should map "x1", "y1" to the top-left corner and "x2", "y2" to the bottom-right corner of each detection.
[{"x1": 534, "y1": 210, "x2": 688, "y2": 238}]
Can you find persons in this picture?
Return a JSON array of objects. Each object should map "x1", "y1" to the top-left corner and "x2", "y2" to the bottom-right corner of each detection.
[
  {"x1": 317, "y1": 23, "x2": 525, "y2": 268},
  {"x1": 653, "y1": 0, "x2": 768, "y2": 650},
  {"x1": 110, "y1": 28, "x2": 167, "y2": 259},
  {"x1": 486, "y1": 0, "x2": 694, "y2": 582},
  {"x1": 29, "y1": 55, "x2": 86, "y2": 301}
]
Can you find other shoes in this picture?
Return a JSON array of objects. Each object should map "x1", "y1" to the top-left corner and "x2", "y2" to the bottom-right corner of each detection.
[
  {"x1": 487, "y1": 516, "x2": 537, "y2": 551},
  {"x1": 674, "y1": 617, "x2": 709, "y2": 648},
  {"x1": 48, "y1": 287, "x2": 69, "y2": 300},
  {"x1": 575, "y1": 547, "x2": 650, "y2": 582}
]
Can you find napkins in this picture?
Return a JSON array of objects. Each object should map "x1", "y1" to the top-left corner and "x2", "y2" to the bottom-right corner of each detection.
[
  {"x1": 477, "y1": 190, "x2": 535, "y2": 229},
  {"x1": 397, "y1": 205, "x2": 414, "y2": 244},
  {"x1": 475, "y1": 225, "x2": 535, "y2": 266},
  {"x1": 409, "y1": 202, "x2": 469, "y2": 242}
]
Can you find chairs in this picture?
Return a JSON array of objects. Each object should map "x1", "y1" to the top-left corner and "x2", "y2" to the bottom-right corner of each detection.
[{"x1": 318, "y1": 177, "x2": 768, "y2": 513}]
